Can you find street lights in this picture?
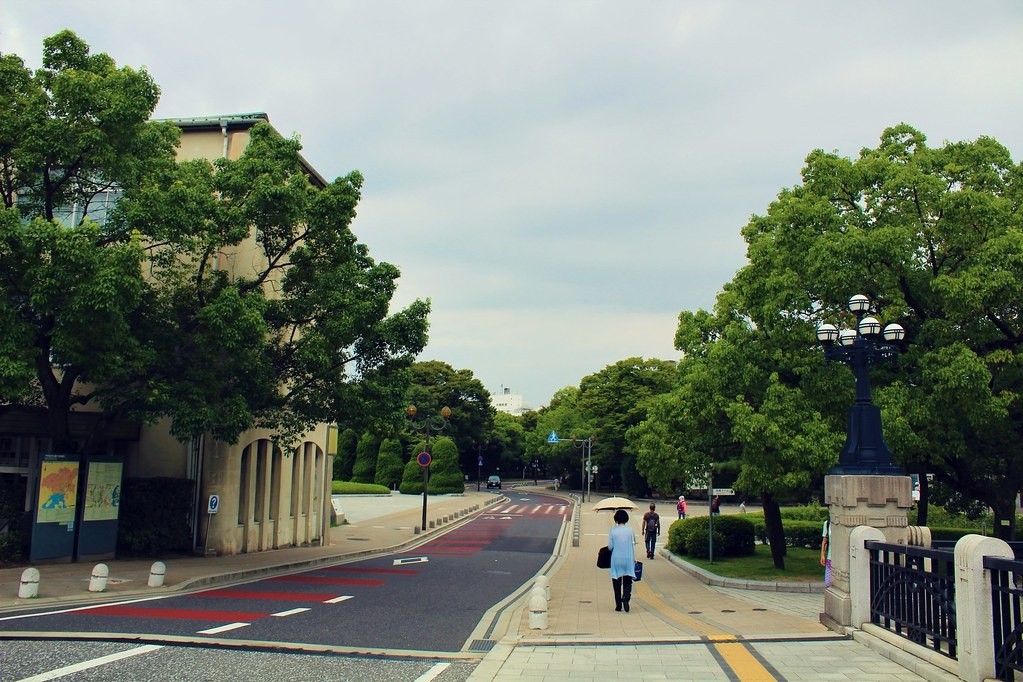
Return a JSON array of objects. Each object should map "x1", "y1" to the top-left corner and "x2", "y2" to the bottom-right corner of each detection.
[
  {"x1": 407, "y1": 404, "x2": 451, "y2": 531},
  {"x1": 572, "y1": 435, "x2": 596, "y2": 503},
  {"x1": 472, "y1": 439, "x2": 489, "y2": 492}
]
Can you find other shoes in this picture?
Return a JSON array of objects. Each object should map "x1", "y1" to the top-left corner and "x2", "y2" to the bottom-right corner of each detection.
[
  {"x1": 615, "y1": 607, "x2": 621, "y2": 611},
  {"x1": 647, "y1": 551, "x2": 654, "y2": 559},
  {"x1": 622, "y1": 597, "x2": 629, "y2": 612}
]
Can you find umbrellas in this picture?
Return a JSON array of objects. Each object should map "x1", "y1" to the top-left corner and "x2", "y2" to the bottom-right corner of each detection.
[{"x1": 593, "y1": 495, "x2": 638, "y2": 523}]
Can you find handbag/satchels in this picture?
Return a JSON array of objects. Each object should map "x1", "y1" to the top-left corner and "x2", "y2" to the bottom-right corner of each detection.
[
  {"x1": 597, "y1": 547, "x2": 612, "y2": 568},
  {"x1": 631, "y1": 561, "x2": 643, "y2": 581}
]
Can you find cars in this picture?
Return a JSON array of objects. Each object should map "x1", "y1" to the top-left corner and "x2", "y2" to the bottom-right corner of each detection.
[{"x1": 487, "y1": 475, "x2": 501, "y2": 489}]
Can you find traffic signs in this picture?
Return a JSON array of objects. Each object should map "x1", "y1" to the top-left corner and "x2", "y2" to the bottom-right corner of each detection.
[{"x1": 713, "y1": 489, "x2": 735, "y2": 495}]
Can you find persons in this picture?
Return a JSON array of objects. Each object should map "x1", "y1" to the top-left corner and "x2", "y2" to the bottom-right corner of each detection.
[
  {"x1": 820, "y1": 505, "x2": 831, "y2": 589},
  {"x1": 914, "y1": 482, "x2": 920, "y2": 502},
  {"x1": 712, "y1": 495, "x2": 720, "y2": 515},
  {"x1": 608, "y1": 510, "x2": 636, "y2": 612},
  {"x1": 678, "y1": 496, "x2": 687, "y2": 519},
  {"x1": 642, "y1": 503, "x2": 660, "y2": 559},
  {"x1": 553, "y1": 478, "x2": 559, "y2": 492},
  {"x1": 739, "y1": 500, "x2": 746, "y2": 513}
]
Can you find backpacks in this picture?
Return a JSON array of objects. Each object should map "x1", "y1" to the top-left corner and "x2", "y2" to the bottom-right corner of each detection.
[
  {"x1": 677, "y1": 503, "x2": 684, "y2": 511},
  {"x1": 646, "y1": 513, "x2": 658, "y2": 532}
]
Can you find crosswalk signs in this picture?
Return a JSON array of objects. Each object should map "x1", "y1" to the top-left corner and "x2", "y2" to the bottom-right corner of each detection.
[{"x1": 548, "y1": 430, "x2": 558, "y2": 443}]
